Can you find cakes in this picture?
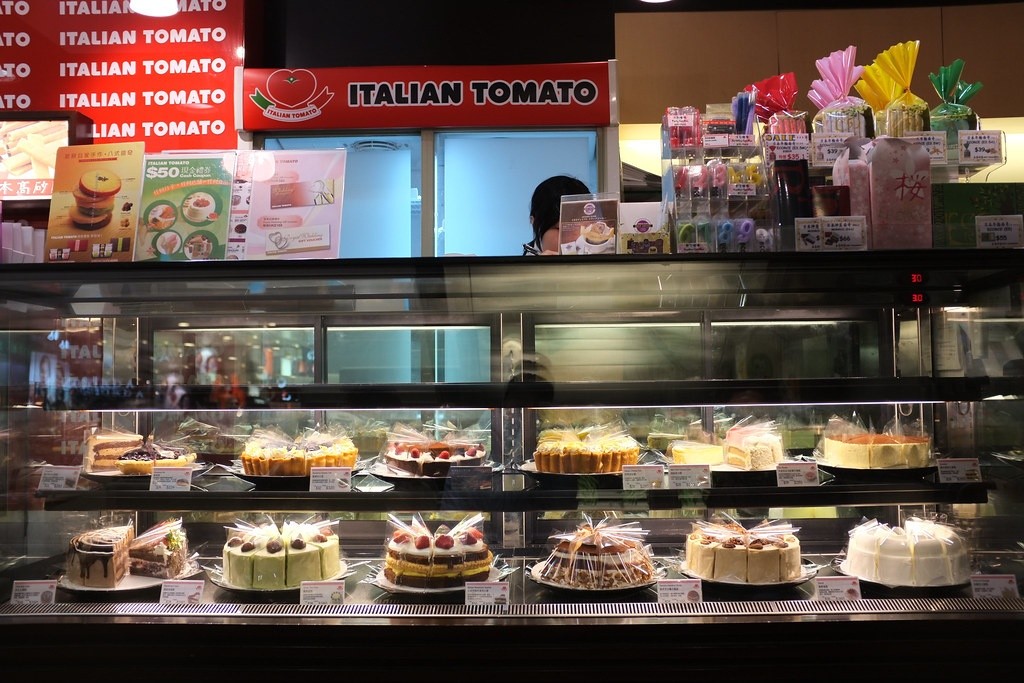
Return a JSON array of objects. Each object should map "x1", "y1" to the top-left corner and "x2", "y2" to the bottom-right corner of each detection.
[
  {"x1": 820, "y1": 431, "x2": 933, "y2": 470},
  {"x1": 240, "y1": 438, "x2": 358, "y2": 476},
  {"x1": 543, "y1": 536, "x2": 654, "y2": 589},
  {"x1": 119, "y1": 444, "x2": 196, "y2": 477},
  {"x1": 843, "y1": 521, "x2": 972, "y2": 586},
  {"x1": 647, "y1": 432, "x2": 687, "y2": 450},
  {"x1": 87, "y1": 432, "x2": 144, "y2": 471},
  {"x1": 685, "y1": 523, "x2": 802, "y2": 584},
  {"x1": 385, "y1": 527, "x2": 493, "y2": 590},
  {"x1": 382, "y1": 442, "x2": 489, "y2": 478},
  {"x1": 222, "y1": 525, "x2": 341, "y2": 589},
  {"x1": 65, "y1": 522, "x2": 186, "y2": 590},
  {"x1": 534, "y1": 435, "x2": 641, "y2": 474},
  {"x1": 186, "y1": 198, "x2": 214, "y2": 220},
  {"x1": 672, "y1": 432, "x2": 782, "y2": 470}
]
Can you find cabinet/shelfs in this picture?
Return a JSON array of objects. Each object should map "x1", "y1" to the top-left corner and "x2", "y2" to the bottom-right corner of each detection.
[{"x1": 0, "y1": 248, "x2": 1024, "y2": 683}]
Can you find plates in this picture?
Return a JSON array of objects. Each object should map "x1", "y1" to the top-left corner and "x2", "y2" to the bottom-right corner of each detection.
[
  {"x1": 59, "y1": 564, "x2": 192, "y2": 598},
  {"x1": 531, "y1": 560, "x2": 669, "y2": 595},
  {"x1": 184, "y1": 241, "x2": 213, "y2": 259},
  {"x1": 510, "y1": 458, "x2": 623, "y2": 489},
  {"x1": 80, "y1": 463, "x2": 214, "y2": 489},
  {"x1": 576, "y1": 235, "x2": 615, "y2": 253},
  {"x1": 227, "y1": 467, "x2": 365, "y2": 491},
  {"x1": 368, "y1": 567, "x2": 501, "y2": 595},
  {"x1": 681, "y1": 558, "x2": 819, "y2": 593},
  {"x1": 802, "y1": 456, "x2": 938, "y2": 483},
  {"x1": 182, "y1": 192, "x2": 216, "y2": 222},
  {"x1": 829, "y1": 555, "x2": 981, "y2": 598},
  {"x1": 156, "y1": 231, "x2": 181, "y2": 255},
  {"x1": 370, "y1": 460, "x2": 505, "y2": 490},
  {"x1": 203, "y1": 560, "x2": 347, "y2": 599},
  {"x1": 148, "y1": 205, "x2": 175, "y2": 231}
]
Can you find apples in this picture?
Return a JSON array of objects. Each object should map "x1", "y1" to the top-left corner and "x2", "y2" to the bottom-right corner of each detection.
[{"x1": 79, "y1": 168, "x2": 122, "y2": 197}]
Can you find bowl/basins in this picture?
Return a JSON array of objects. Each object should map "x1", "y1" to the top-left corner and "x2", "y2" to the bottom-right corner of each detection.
[{"x1": 582, "y1": 234, "x2": 609, "y2": 252}]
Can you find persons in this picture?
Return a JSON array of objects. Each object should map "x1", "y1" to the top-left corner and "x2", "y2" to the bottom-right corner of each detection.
[{"x1": 526, "y1": 175, "x2": 592, "y2": 256}]
